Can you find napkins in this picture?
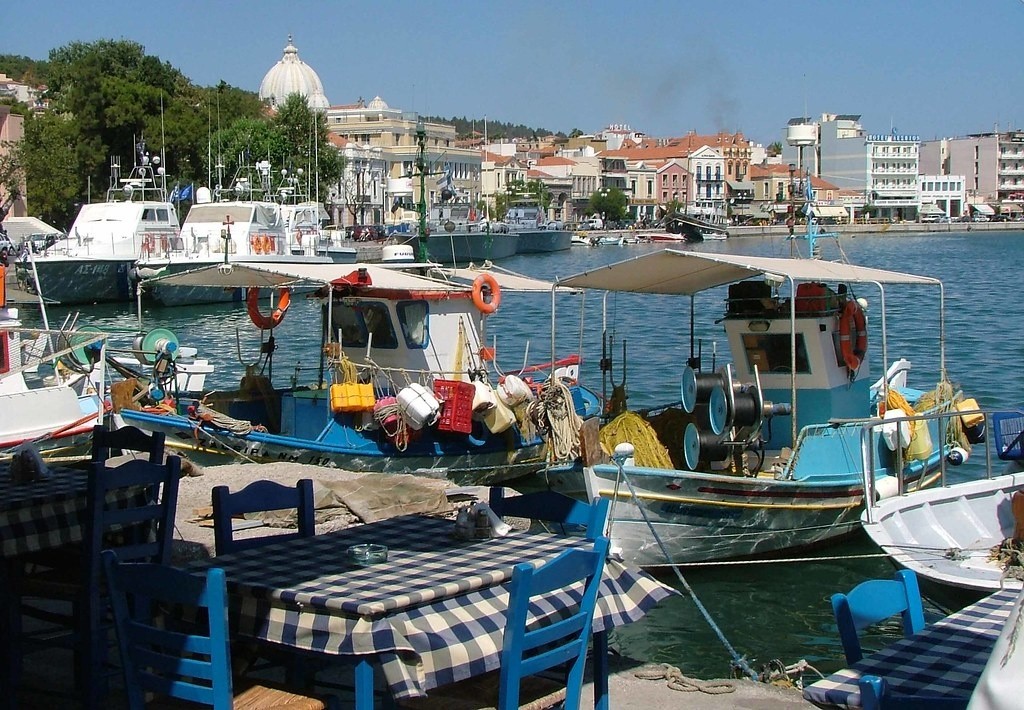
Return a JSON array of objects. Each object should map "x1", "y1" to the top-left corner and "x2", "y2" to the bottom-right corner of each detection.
[{"x1": 475, "y1": 502, "x2": 513, "y2": 537}]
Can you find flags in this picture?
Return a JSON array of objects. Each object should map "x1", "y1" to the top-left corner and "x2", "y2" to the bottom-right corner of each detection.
[{"x1": 179, "y1": 184, "x2": 192, "y2": 201}]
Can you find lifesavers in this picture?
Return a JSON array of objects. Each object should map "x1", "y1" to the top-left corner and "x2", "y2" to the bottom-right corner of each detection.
[
  {"x1": 246, "y1": 287, "x2": 290, "y2": 330},
  {"x1": 253, "y1": 235, "x2": 262, "y2": 254},
  {"x1": 261, "y1": 236, "x2": 271, "y2": 254},
  {"x1": 145, "y1": 232, "x2": 155, "y2": 253},
  {"x1": 839, "y1": 299, "x2": 869, "y2": 372},
  {"x1": 472, "y1": 275, "x2": 502, "y2": 315}
]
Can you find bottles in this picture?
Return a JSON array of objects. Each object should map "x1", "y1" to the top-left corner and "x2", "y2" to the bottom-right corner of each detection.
[
  {"x1": 11, "y1": 450, "x2": 40, "y2": 483},
  {"x1": 456, "y1": 501, "x2": 489, "y2": 538}
]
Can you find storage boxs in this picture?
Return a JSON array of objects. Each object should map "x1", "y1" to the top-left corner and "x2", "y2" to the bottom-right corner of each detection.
[{"x1": 434, "y1": 379, "x2": 476, "y2": 433}]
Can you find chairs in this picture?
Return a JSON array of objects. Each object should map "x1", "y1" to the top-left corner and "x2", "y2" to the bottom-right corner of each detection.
[
  {"x1": 34, "y1": 425, "x2": 181, "y2": 710},
  {"x1": 106, "y1": 550, "x2": 339, "y2": 710},
  {"x1": 213, "y1": 478, "x2": 318, "y2": 556},
  {"x1": 397, "y1": 537, "x2": 610, "y2": 710},
  {"x1": 489, "y1": 488, "x2": 609, "y2": 543},
  {"x1": 831, "y1": 569, "x2": 924, "y2": 665}
]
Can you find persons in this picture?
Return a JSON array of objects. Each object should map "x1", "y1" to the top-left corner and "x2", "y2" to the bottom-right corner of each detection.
[{"x1": 358, "y1": 228, "x2": 372, "y2": 242}]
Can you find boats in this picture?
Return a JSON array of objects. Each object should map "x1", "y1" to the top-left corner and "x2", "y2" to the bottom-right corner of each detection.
[
  {"x1": 18, "y1": 90, "x2": 360, "y2": 303},
  {"x1": 391, "y1": 116, "x2": 516, "y2": 262},
  {"x1": 848, "y1": 410, "x2": 1024, "y2": 607},
  {"x1": 104, "y1": 260, "x2": 609, "y2": 486},
  {"x1": 0, "y1": 242, "x2": 214, "y2": 465},
  {"x1": 543, "y1": 167, "x2": 953, "y2": 568},
  {"x1": 505, "y1": 223, "x2": 574, "y2": 253},
  {"x1": 572, "y1": 234, "x2": 682, "y2": 244}
]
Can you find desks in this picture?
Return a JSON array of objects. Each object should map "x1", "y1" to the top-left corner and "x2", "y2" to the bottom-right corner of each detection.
[
  {"x1": 0, "y1": 459, "x2": 153, "y2": 710},
  {"x1": 802, "y1": 588, "x2": 1022, "y2": 709},
  {"x1": 159, "y1": 511, "x2": 684, "y2": 710}
]
